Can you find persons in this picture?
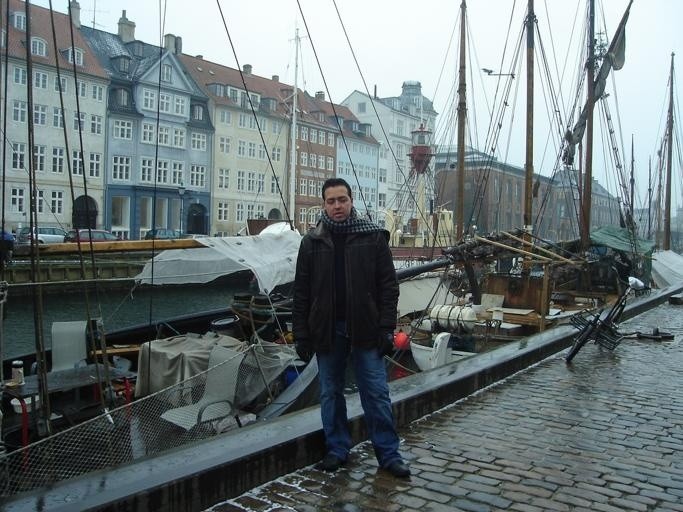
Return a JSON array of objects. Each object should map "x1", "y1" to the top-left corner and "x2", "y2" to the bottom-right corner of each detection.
[{"x1": 292, "y1": 178, "x2": 412, "y2": 478}]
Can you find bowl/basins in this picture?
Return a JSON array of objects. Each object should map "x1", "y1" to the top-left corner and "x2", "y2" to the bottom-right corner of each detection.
[
  {"x1": 5, "y1": 382, "x2": 19, "y2": 389},
  {"x1": 10, "y1": 396, "x2": 40, "y2": 414}
]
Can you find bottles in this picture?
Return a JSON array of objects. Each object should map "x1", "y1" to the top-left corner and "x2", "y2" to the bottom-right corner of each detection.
[{"x1": 491, "y1": 309, "x2": 504, "y2": 327}]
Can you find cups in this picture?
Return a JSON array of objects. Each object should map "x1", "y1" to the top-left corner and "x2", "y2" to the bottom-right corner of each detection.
[{"x1": 11, "y1": 360, "x2": 24, "y2": 384}]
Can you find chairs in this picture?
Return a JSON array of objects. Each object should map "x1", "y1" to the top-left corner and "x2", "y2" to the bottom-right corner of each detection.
[{"x1": 158, "y1": 344, "x2": 249, "y2": 441}]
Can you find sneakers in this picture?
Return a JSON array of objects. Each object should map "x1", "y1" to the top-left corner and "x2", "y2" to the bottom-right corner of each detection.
[
  {"x1": 387, "y1": 458, "x2": 411, "y2": 477},
  {"x1": 322, "y1": 453, "x2": 346, "y2": 471}
]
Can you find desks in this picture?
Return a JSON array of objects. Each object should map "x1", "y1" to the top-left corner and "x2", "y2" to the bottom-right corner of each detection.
[{"x1": 0, "y1": 363, "x2": 127, "y2": 467}]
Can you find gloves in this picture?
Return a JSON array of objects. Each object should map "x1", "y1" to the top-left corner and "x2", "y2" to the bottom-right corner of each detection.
[
  {"x1": 375, "y1": 328, "x2": 394, "y2": 357},
  {"x1": 295, "y1": 346, "x2": 313, "y2": 363}
]
